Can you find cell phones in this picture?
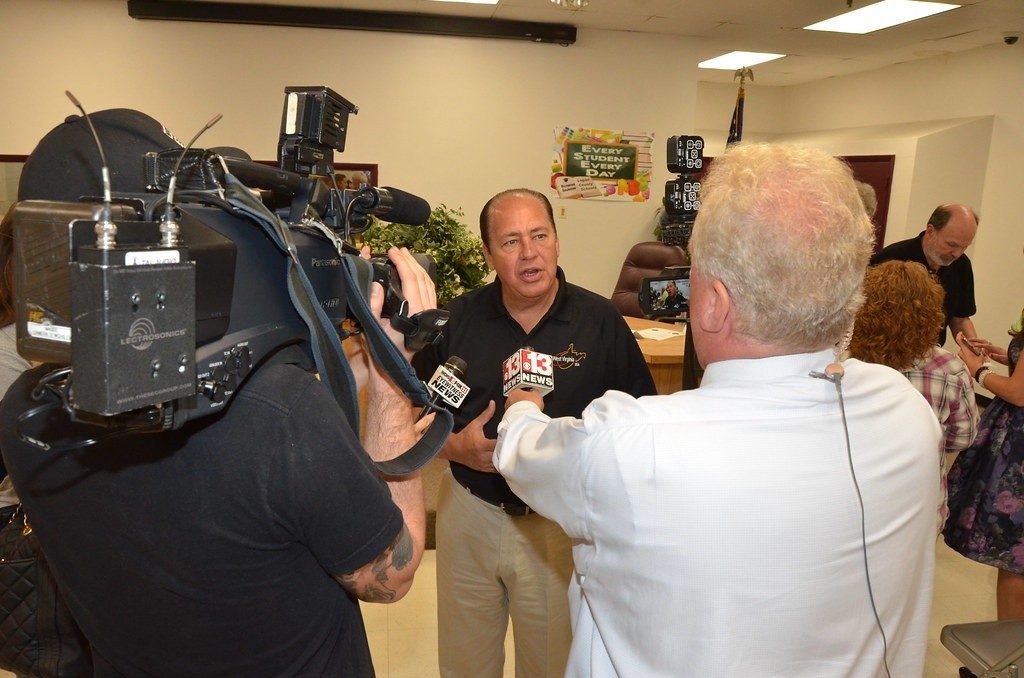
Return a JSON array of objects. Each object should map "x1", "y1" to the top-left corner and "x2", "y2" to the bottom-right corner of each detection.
[{"x1": 963, "y1": 338, "x2": 981, "y2": 357}]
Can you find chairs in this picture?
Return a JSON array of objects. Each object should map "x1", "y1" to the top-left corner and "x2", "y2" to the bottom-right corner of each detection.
[
  {"x1": 940, "y1": 618, "x2": 1024, "y2": 678},
  {"x1": 609, "y1": 241, "x2": 689, "y2": 327}
]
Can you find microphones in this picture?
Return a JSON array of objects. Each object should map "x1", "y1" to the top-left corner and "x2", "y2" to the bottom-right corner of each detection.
[
  {"x1": 357, "y1": 186, "x2": 431, "y2": 226},
  {"x1": 415, "y1": 355, "x2": 471, "y2": 424},
  {"x1": 503, "y1": 346, "x2": 554, "y2": 397}
]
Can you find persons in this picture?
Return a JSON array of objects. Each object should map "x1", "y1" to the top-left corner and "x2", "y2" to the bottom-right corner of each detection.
[
  {"x1": 664, "y1": 280, "x2": 686, "y2": 308},
  {"x1": 334, "y1": 170, "x2": 368, "y2": 191},
  {"x1": 940, "y1": 308, "x2": 1024, "y2": 620},
  {"x1": 411, "y1": 189, "x2": 656, "y2": 678},
  {"x1": 868, "y1": 204, "x2": 990, "y2": 366},
  {"x1": 492, "y1": 142, "x2": 944, "y2": 677},
  {"x1": 836, "y1": 260, "x2": 982, "y2": 544},
  {"x1": 0, "y1": 108, "x2": 437, "y2": 678}
]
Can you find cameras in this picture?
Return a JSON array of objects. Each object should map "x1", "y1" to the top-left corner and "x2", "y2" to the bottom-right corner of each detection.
[{"x1": 639, "y1": 265, "x2": 693, "y2": 315}]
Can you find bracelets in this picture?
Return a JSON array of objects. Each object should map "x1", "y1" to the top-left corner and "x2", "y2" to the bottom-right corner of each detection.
[
  {"x1": 975, "y1": 366, "x2": 989, "y2": 383},
  {"x1": 979, "y1": 370, "x2": 992, "y2": 387}
]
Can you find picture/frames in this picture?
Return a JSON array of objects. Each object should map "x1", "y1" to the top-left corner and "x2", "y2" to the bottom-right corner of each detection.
[{"x1": 253, "y1": 161, "x2": 378, "y2": 192}]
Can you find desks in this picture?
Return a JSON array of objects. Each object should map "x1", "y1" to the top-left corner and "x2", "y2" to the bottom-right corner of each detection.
[{"x1": 622, "y1": 315, "x2": 687, "y2": 395}]
